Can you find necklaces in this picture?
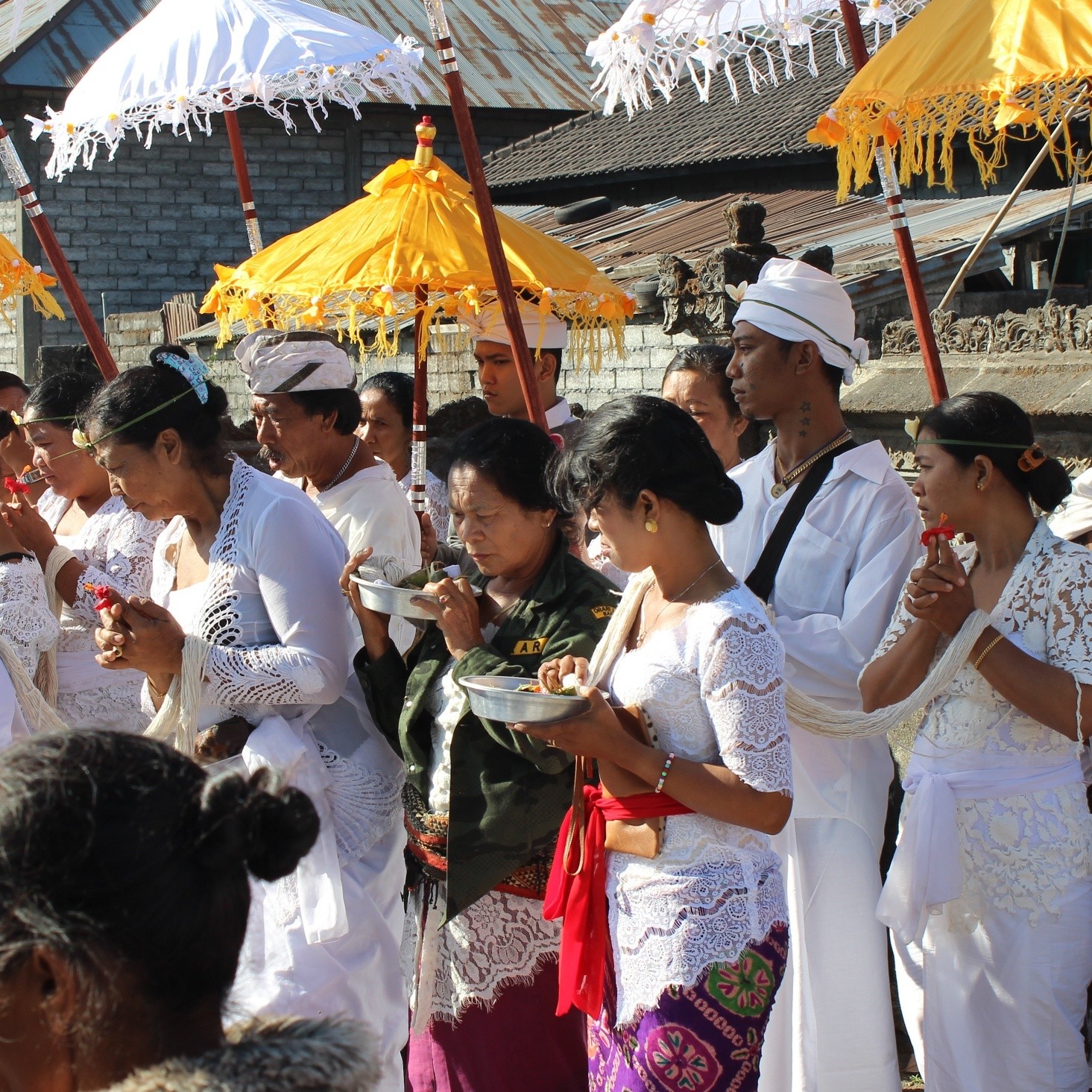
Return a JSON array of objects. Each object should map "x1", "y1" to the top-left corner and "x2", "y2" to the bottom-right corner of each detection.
[
  {"x1": 319, "y1": 435, "x2": 362, "y2": 494},
  {"x1": 635, "y1": 556, "x2": 723, "y2": 643},
  {"x1": 770, "y1": 423, "x2": 854, "y2": 499}
]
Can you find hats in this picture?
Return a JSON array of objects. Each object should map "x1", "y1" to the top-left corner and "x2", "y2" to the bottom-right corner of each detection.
[
  {"x1": 234, "y1": 328, "x2": 358, "y2": 394},
  {"x1": 470, "y1": 296, "x2": 568, "y2": 349},
  {"x1": 726, "y1": 257, "x2": 871, "y2": 385}
]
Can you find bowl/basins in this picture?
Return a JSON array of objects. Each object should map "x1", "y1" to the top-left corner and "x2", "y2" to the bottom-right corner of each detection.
[
  {"x1": 349, "y1": 571, "x2": 483, "y2": 620},
  {"x1": 458, "y1": 675, "x2": 610, "y2": 725}
]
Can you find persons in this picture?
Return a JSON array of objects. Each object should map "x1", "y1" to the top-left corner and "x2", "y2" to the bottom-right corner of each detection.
[
  {"x1": 80, "y1": 344, "x2": 413, "y2": 1092},
  {"x1": 339, "y1": 420, "x2": 624, "y2": 1092},
  {"x1": 0, "y1": 244, "x2": 948, "y2": 1092},
  {"x1": 857, "y1": 392, "x2": 1092, "y2": 1092},
  {"x1": 545, "y1": 398, "x2": 795, "y2": 1092},
  {"x1": 0, "y1": 726, "x2": 390, "y2": 1092}
]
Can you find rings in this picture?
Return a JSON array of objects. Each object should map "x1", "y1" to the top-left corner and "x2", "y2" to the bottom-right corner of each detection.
[
  {"x1": 547, "y1": 740, "x2": 561, "y2": 750},
  {"x1": 544, "y1": 665, "x2": 561, "y2": 671},
  {"x1": 439, "y1": 593, "x2": 451, "y2": 605},
  {"x1": 340, "y1": 587, "x2": 350, "y2": 596}
]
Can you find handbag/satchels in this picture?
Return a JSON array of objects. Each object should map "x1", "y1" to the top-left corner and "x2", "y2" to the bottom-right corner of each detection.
[{"x1": 596, "y1": 705, "x2": 666, "y2": 858}]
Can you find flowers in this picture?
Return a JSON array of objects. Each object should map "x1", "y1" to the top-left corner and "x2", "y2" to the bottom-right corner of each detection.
[
  {"x1": 83, "y1": 582, "x2": 114, "y2": 612},
  {"x1": 5, "y1": 465, "x2": 32, "y2": 495},
  {"x1": 72, "y1": 428, "x2": 91, "y2": 450},
  {"x1": 725, "y1": 280, "x2": 747, "y2": 302},
  {"x1": 10, "y1": 410, "x2": 23, "y2": 426},
  {"x1": 918, "y1": 512, "x2": 955, "y2": 547},
  {"x1": 904, "y1": 416, "x2": 920, "y2": 441}
]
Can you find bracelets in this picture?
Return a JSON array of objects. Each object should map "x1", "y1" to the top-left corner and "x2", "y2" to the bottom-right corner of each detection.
[
  {"x1": 972, "y1": 633, "x2": 1009, "y2": 673},
  {"x1": 655, "y1": 752, "x2": 678, "y2": 793}
]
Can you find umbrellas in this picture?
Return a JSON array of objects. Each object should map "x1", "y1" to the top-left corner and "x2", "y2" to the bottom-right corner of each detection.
[
  {"x1": 585, "y1": 0, "x2": 1092, "y2": 396},
  {"x1": 0, "y1": 230, "x2": 65, "y2": 328},
  {"x1": 200, "y1": 112, "x2": 636, "y2": 522},
  {"x1": 23, "y1": 0, "x2": 423, "y2": 327}
]
